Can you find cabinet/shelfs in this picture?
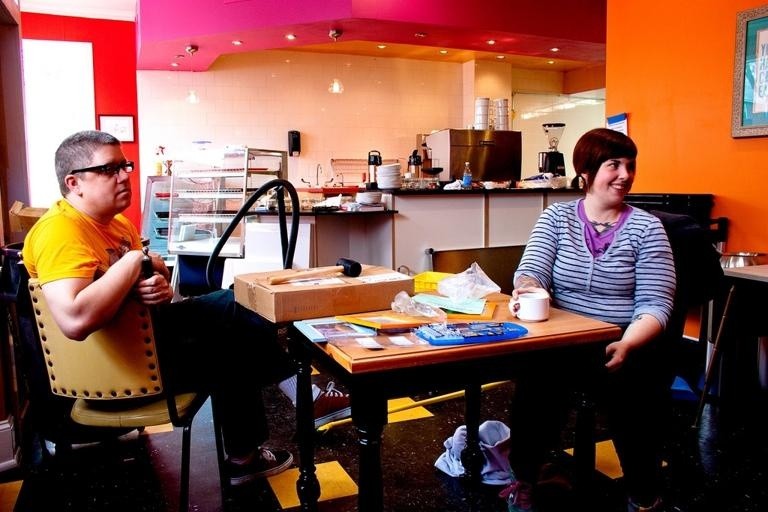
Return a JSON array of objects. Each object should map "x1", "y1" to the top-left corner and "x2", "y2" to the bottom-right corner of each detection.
[
  {"x1": 139, "y1": 169, "x2": 227, "y2": 268},
  {"x1": 166, "y1": 141, "x2": 290, "y2": 260}
]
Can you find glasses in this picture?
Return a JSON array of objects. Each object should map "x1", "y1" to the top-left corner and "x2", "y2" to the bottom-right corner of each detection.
[{"x1": 71, "y1": 160, "x2": 136, "y2": 175}]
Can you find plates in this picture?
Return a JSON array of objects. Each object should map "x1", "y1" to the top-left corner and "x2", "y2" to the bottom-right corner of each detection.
[
  {"x1": 356, "y1": 163, "x2": 402, "y2": 207},
  {"x1": 420, "y1": 168, "x2": 443, "y2": 175}
]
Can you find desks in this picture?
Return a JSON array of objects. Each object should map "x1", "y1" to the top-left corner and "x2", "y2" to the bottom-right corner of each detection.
[
  {"x1": 265, "y1": 262, "x2": 623, "y2": 509},
  {"x1": 723, "y1": 258, "x2": 766, "y2": 511}
]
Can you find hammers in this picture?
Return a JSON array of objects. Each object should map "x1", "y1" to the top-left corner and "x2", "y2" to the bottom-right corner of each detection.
[{"x1": 267, "y1": 257, "x2": 362, "y2": 285}]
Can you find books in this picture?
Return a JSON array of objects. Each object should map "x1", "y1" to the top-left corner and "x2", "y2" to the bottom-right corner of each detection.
[
  {"x1": 337, "y1": 310, "x2": 432, "y2": 329},
  {"x1": 292, "y1": 316, "x2": 378, "y2": 343},
  {"x1": 439, "y1": 302, "x2": 499, "y2": 320}
]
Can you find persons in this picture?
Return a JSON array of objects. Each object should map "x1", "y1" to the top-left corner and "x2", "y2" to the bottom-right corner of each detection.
[
  {"x1": 511, "y1": 128, "x2": 676, "y2": 509},
  {"x1": 22, "y1": 130, "x2": 352, "y2": 486}
]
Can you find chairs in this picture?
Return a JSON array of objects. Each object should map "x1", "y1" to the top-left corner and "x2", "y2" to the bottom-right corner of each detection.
[
  {"x1": 1, "y1": 239, "x2": 145, "y2": 507},
  {"x1": 28, "y1": 243, "x2": 235, "y2": 510},
  {"x1": 624, "y1": 188, "x2": 732, "y2": 398},
  {"x1": 538, "y1": 206, "x2": 728, "y2": 512}
]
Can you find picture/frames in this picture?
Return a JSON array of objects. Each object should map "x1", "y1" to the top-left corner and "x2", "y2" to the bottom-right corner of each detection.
[
  {"x1": 97, "y1": 113, "x2": 136, "y2": 146},
  {"x1": 728, "y1": 3, "x2": 767, "y2": 140}
]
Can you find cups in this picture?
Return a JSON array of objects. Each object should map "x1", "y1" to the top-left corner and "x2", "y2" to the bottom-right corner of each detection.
[
  {"x1": 510, "y1": 293, "x2": 549, "y2": 323},
  {"x1": 425, "y1": 174, "x2": 440, "y2": 190},
  {"x1": 472, "y1": 97, "x2": 509, "y2": 132}
]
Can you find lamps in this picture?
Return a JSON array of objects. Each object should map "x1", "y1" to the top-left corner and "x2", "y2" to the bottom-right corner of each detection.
[
  {"x1": 186, "y1": 43, "x2": 199, "y2": 55},
  {"x1": 327, "y1": 28, "x2": 344, "y2": 43}
]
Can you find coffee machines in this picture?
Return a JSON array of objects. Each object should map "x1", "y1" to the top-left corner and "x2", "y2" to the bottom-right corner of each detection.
[
  {"x1": 425, "y1": 129, "x2": 522, "y2": 187},
  {"x1": 537, "y1": 123, "x2": 566, "y2": 178}
]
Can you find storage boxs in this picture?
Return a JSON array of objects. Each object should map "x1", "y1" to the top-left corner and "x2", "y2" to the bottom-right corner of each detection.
[{"x1": 232, "y1": 255, "x2": 417, "y2": 324}]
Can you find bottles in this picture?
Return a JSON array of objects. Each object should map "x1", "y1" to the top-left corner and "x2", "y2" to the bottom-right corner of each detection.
[
  {"x1": 154, "y1": 153, "x2": 163, "y2": 177},
  {"x1": 367, "y1": 154, "x2": 381, "y2": 188},
  {"x1": 408, "y1": 149, "x2": 422, "y2": 181},
  {"x1": 462, "y1": 161, "x2": 474, "y2": 191}
]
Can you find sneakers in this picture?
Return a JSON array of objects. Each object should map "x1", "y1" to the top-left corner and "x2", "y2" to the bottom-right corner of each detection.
[
  {"x1": 313, "y1": 386, "x2": 352, "y2": 426},
  {"x1": 224, "y1": 446, "x2": 293, "y2": 485},
  {"x1": 507, "y1": 479, "x2": 533, "y2": 512}
]
